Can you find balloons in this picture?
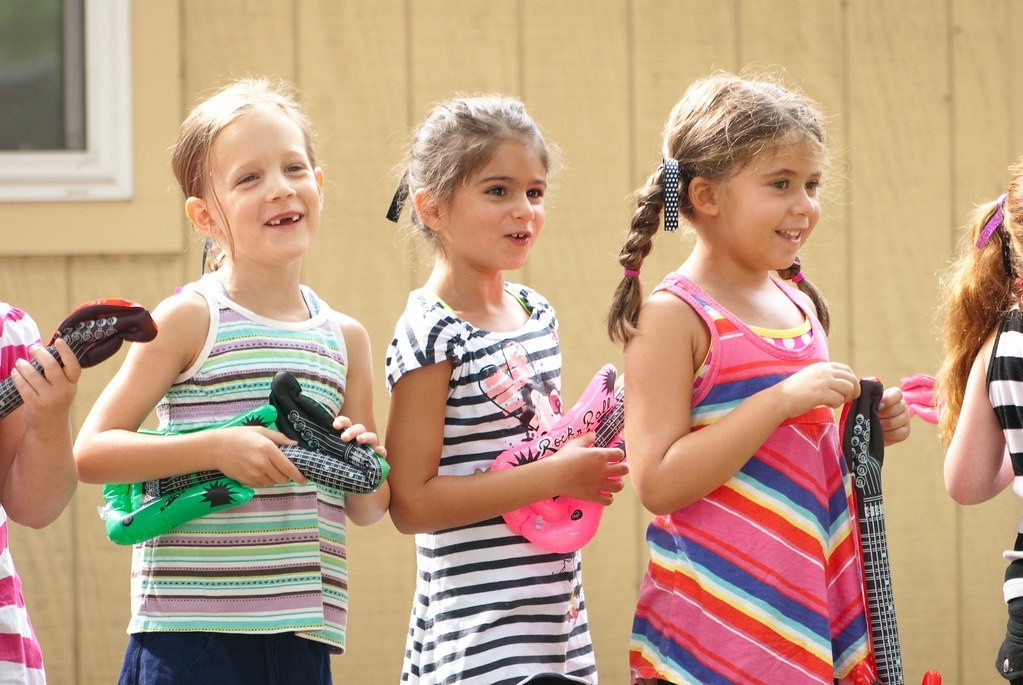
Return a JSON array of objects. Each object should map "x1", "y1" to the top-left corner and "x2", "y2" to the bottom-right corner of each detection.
[
  {"x1": 98, "y1": 370, "x2": 391, "y2": 543},
  {"x1": 490, "y1": 363, "x2": 629, "y2": 552},
  {"x1": 838, "y1": 374, "x2": 943, "y2": 685},
  {"x1": 900, "y1": 373, "x2": 945, "y2": 425},
  {"x1": 0, "y1": 295, "x2": 158, "y2": 422}
]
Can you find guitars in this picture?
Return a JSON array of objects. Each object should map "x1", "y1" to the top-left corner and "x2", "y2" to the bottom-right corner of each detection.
[
  {"x1": 0, "y1": 296, "x2": 158, "y2": 426},
  {"x1": 100, "y1": 370, "x2": 391, "y2": 548},
  {"x1": 836, "y1": 374, "x2": 944, "y2": 685},
  {"x1": 491, "y1": 362, "x2": 627, "y2": 554}
]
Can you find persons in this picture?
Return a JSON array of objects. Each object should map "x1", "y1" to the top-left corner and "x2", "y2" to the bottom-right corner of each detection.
[
  {"x1": 383, "y1": 97, "x2": 628, "y2": 685},
  {"x1": 605, "y1": 73, "x2": 912, "y2": 685},
  {"x1": 73, "y1": 76, "x2": 395, "y2": 685},
  {"x1": 0, "y1": 300, "x2": 82, "y2": 685},
  {"x1": 933, "y1": 159, "x2": 1023, "y2": 685}
]
